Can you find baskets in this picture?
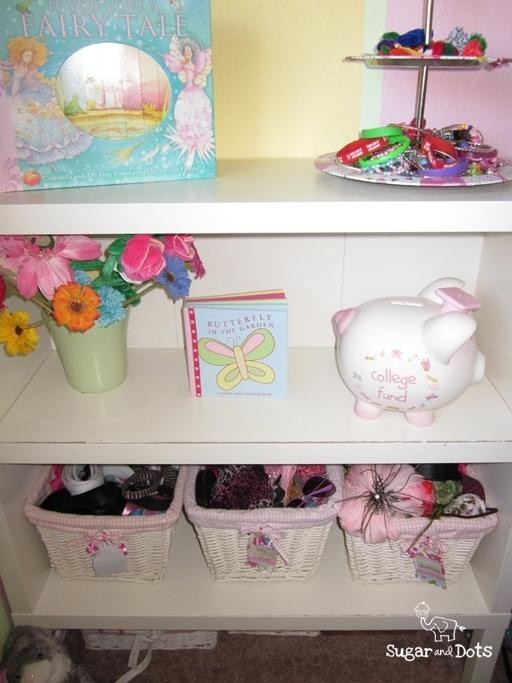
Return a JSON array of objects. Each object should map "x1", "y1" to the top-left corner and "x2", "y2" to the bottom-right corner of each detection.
[
  {"x1": 80, "y1": 628, "x2": 219, "y2": 651},
  {"x1": 337, "y1": 466, "x2": 500, "y2": 587},
  {"x1": 23, "y1": 464, "x2": 187, "y2": 582},
  {"x1": 186, "y1": 466, "x2": 342, "y2": 581}
]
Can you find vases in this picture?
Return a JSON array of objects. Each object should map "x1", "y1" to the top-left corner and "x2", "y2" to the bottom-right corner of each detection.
[{"x1": 40, "y1": 313, "x2": 129, "y2": 393}]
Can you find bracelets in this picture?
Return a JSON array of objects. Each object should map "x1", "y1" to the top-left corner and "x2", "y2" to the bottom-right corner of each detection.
[{"x1": 336, "y1": 123, "x2": 498, "y2": 178}]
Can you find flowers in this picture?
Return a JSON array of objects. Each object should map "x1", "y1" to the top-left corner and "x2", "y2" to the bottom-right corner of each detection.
[{"x1": 0, "y1": 234, "x2": 207, "y2": 356}]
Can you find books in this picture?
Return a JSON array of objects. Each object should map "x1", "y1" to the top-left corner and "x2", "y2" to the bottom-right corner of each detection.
[{"x1": 182, "y1": 289, "x2": 289, "y2": 398}]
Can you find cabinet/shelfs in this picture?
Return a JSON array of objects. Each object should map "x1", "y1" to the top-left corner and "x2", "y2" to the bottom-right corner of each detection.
[{"x1": 0, "y1": 157, "x2": 512, "y2": 683}]
